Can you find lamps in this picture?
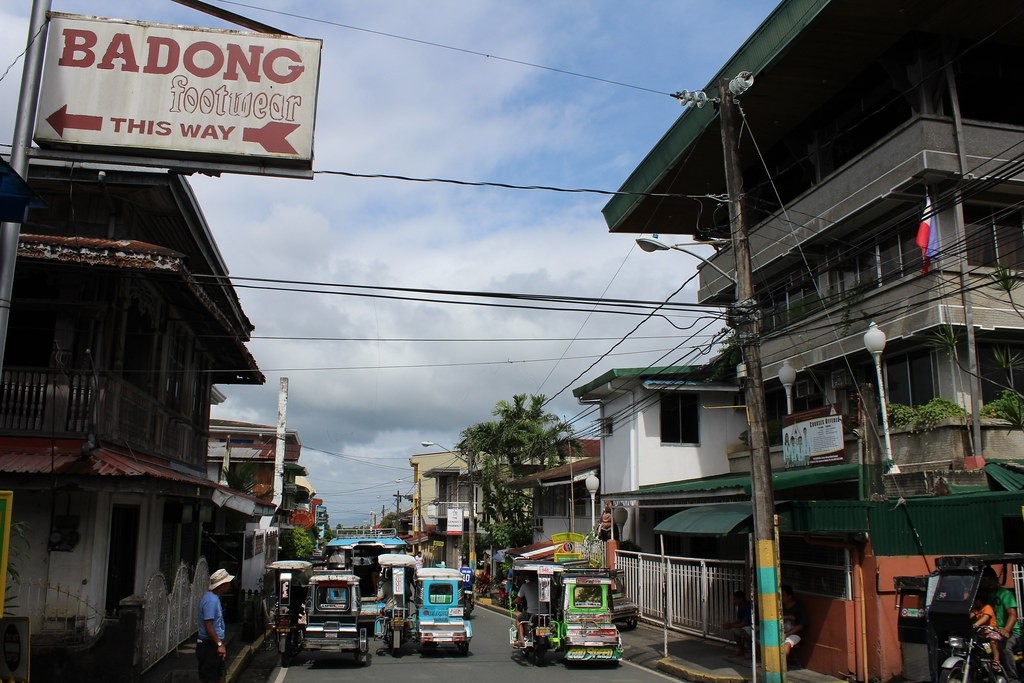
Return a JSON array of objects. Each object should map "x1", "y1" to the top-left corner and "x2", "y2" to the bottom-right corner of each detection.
[
  {"x1": 864, "y1": 322, "x2": 886, "y2": 354},
  {"x1": 778, "y1": 361, "x2": 796, "y2": 386}
]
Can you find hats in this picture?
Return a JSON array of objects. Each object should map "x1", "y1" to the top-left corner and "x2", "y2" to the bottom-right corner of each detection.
[{"x1": 208, "y1": 569, "x2": 235, "y2": 591}]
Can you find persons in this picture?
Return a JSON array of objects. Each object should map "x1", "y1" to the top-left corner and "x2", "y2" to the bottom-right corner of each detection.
[
  {"x1": 375, "y1": 569, "x2": 413, "y2": 638},
  {"x1": 784, "y1": 428, "x2": 809, "y2": 466},
  {"x1": 976, "y1": 567, "x2": 1021, "y2": 683},
  {"x1": 969, "y1": 593, "x2": 1001, "y2": 671},
  {"x1": 506, "y1": 569, "x2": 514, "y2": 599},
  {"x1": 782, "y1": 585, "x2": 806, "y2": 656},
  {"x1": 596, "y1": 501, "x2": 611, "y2": 542},
  {"x1": 722, "y1": 591, "x2": 761, "y2": 659},
  {"x1": 196, "y1": 569, "x2": 235, "y2": 683},
  {"x1": 512, "y1": 574, "x2": 547, "y2": 647},
  {"x1": 459, "y1": 561, "x2": 475, "y2": 608},
  {"x1": 415, "y1": 552, "x2": 425, "y2": 567}
]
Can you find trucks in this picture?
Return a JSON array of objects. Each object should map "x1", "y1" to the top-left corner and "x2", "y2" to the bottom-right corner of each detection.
[{"x1": 326, "y1": 528, "x2": 408, "y2": 623}]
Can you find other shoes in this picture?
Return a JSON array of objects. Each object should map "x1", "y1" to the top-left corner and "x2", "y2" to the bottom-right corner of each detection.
[
  {"x1": 991, "y1": 660, "x2": 1003, "y2": 673},
  {"x1": 514, "y1": 641, "x2": 525, "y2": 646}
]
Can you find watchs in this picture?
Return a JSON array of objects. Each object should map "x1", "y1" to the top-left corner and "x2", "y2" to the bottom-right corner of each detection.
[{"x1": 217, "y1": 641, "x2": 222, "y2": 647}]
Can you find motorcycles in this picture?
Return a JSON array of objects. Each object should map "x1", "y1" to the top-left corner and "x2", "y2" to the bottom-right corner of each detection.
[{"x1": 462, "y1": 585, "x2": 473, "y2": 619}]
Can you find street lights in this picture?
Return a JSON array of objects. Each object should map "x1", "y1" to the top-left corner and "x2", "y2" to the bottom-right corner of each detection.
[{"x1": 421, "y1": 440, "x2": 476, "y2": 596}]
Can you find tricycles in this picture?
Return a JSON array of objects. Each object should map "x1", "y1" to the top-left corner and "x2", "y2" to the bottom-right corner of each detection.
[
  {"x1": 266, "y1": 560, "x2": 368, "y2": 668},
  {"x1": 894, "y1": 555, "x2": 1024, "y2": 683},
  {"x1": 378, "y1": 554, "x2": 472, "y2": 657},
  {"x1": 508, "y1": 558, "x2": 623, "y2": 666}
]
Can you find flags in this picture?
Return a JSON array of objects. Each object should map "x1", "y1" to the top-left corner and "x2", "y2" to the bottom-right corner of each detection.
[{"x1": 915, "y1": 196, "x2": 941, "y2": 276}]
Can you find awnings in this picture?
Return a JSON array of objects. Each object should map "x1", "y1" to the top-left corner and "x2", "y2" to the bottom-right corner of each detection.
[
  {"x1": 406, "y1": 534, "x2": 428, "y2": 544},
  {"x1": 600, "y1": 464, "x2": 859, "y2": 500},
  {"x1": 656, "y1": 499, "x2": 865, "y2": 538}
]
